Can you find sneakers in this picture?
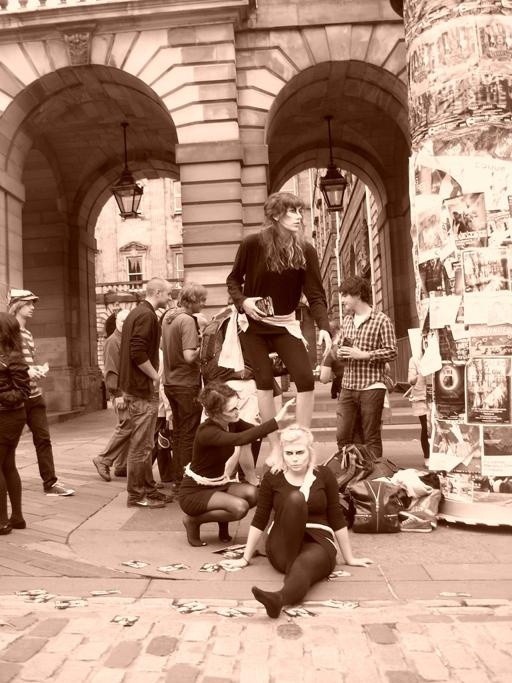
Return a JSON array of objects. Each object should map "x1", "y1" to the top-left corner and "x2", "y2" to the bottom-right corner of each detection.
[
  {"x1": 155, "y1": 482, "x2": 178, "y2": 492},
  {"x1": 399, "y1": 517, "x2": 433, "y2": 532},
  {"x1": 399, "y1": 509, "x2": 437, "y2": 528},
  {"x1": 114, "y1": 469, "x2": 127, "y2": 477},
  {"x1": 93, "y1": 457, "x2": 111, "y2": 481},
  {"x1": 46, "y1": 483, "x2": 75, "y2": 496}
]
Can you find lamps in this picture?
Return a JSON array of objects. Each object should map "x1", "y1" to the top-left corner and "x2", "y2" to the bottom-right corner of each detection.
[
  {"x1": 111, "y1": 122, "x2": 143, "y2": 219},
  {"x1": 318, "y1": 115, "x2": 348, "y2": 213}
]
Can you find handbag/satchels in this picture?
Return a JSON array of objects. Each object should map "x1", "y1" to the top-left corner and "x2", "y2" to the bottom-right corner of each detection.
[{"x1": 319, "y1": 443, "x2": 412, "y2": 533}]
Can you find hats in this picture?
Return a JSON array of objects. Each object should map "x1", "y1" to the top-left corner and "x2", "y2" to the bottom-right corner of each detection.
[{"x1": 6, "y1": 289, "x2": 38, "y2": 307}]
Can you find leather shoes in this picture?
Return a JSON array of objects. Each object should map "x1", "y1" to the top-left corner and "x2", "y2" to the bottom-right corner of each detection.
[
  {"x1": 127, "y1": 494, "x2": 165, "y2": 507},
  {"x1": 9, "y1": 516, "x2": 26, "y2": 529},
  {"x1": 146, "y1": 489, "x2": 174, "y2": 502},
  {"x1": 0, "y1": 522, "x2": 12, "y2": 535}
]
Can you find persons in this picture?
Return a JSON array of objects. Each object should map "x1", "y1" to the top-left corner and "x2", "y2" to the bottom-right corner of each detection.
[
  {"x1": 91, "y1": 188, "x2": 429, "y2": 621},
  {"x1": 0, "y1": 289, "x2": 77, "y2": 536}
]
